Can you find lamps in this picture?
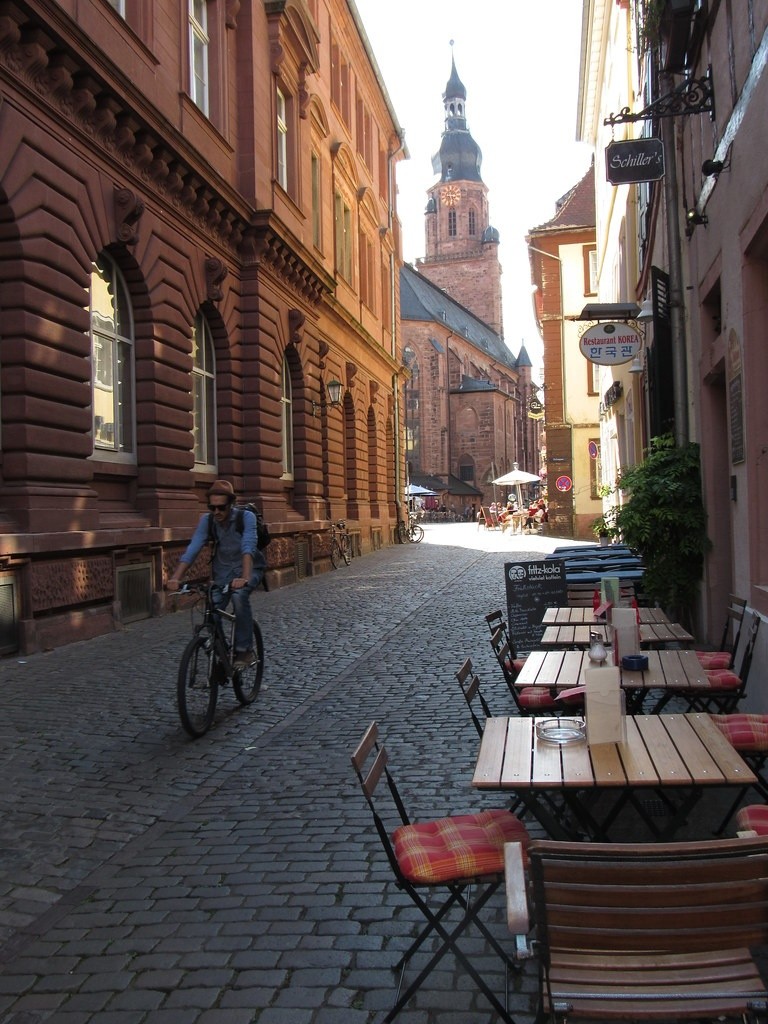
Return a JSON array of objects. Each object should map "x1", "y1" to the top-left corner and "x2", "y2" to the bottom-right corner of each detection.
[
  {"x1": 685, "y1": 208, "x2": 704, "y2": 225},
  {"x1": 312, "y1": 376, "x2": 345, "y2": 418},
  {"x1": 635, "y1": 289, "x2": 653, "y2": 323},
  {"x1": 628, "y1": 350, "x2": 647, "y2": 374},
  {"x1": 701, "y1": 159, "x2": 723, "y2": 176}
]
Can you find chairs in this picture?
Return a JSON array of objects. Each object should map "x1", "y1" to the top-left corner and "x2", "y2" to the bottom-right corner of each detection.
[
  {"x1": 349, "y1": 719, "x2": 531, "y2": 1024},
  {"x1": 498, "y1": 512, "x2": 511, "y2": 534},
  {"x1": 485, "y1": 609, "x2": 527, "y2": 683},
  {"x1": 674, "y1": 610, "x2": 761, "y2": 715},
  {"x1": 502, "y1": 830, "x2": 768, "y2": 1024},
  {"x1": 566, "y1": 581, "x2": 636, "y2": 607},
  {"x1": 532, "y1": 513, "x2": 547, "y2": 532},
  {"x1": 490, "y1": 628, "x2": 585, "y2": 716},
  {"x1": 707, "y1": 712, "x2": 768, "y2": 836},
  {"x1": 678, "y1": 593, "x2": 748, "y2": 712},
  {"x1": 455, "y1": 658, "x2": 571, "y2": 828}
]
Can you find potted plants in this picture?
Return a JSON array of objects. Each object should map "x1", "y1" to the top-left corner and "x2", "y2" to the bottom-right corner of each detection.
[{"x1": 587, "y1": 515, "x2": 619, "y2": 545}]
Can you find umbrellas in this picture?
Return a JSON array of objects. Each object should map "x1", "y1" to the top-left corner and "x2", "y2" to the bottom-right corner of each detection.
[
  {"x1": 405, "y1": 483, "x2": 433, "y2": 511},
  {"x1": 414, "y1": 486, "x2": 440, "y2": 508},
  {"x1": 492, "y1": 469, "x2": 542, "y2": 507}
]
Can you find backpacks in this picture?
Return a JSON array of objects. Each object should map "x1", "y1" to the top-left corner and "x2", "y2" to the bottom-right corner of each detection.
[{"x1": 203, "y1": 503, "x2": 271, "y2": 551}]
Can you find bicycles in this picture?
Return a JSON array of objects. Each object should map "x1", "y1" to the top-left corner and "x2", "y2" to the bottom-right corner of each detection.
[
  {"x1": 162, "y1": 581, "x2": 264, "y2": 739},
  {"x1": 330, "y1": 522, "x2": 352, "y2": 569},
  {"x1": 398, "y1": 508, "x2": 425, "y2": 544}
]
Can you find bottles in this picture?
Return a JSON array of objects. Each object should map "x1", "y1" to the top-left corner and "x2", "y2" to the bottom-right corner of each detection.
[
  {"x1": 588, "y1": 633, "x2": 607, "y2": 662},
  {"x1": 593, "y1": 589, "x2": 601, "y2": 612}
]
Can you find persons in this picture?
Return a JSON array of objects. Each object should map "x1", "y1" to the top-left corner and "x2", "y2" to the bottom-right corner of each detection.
[
  {"x1": 465, "y1": 506, "x2": 471, "y2": 519},
  {"x1": 471, "y1": 507, "x2": 475, "y2": 521},
  {"x1": 440, "y1": 504, "x2": 446, "y2": 512},
  {"x1": 489, "y1": 502, "x2": 513, "y2": 511},
  {"x1": 524, "y1": 499, "x2": 545, "y2": 529},
  {"x1": 167, "y1": 480, "x2": 265, "y2": 691},
  {"x1": 448, "y1": 505, "x2": 456, "y2": 512}
]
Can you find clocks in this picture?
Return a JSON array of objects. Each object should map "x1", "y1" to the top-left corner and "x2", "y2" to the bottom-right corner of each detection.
[{"x1": 441, "y1": 185, "x2": 461, "y2": 206}]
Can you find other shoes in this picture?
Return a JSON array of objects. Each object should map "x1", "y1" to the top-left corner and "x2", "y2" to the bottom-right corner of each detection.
[
  {"x1": 232, "y1": 647, "x2": 251, "y2": 672},
  {"x1": 522, "y1": 526, "x2": 526, "y2": 529}
]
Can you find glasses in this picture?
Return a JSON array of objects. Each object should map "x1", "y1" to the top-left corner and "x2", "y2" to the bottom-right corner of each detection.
[{"x1": 207, "y1": 501, "x2": 231, "y2": 512}]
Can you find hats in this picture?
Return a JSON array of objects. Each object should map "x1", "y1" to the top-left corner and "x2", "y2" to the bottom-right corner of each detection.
[{"x1": 205, "y1": 480, "x2": 237, "y2": 501}]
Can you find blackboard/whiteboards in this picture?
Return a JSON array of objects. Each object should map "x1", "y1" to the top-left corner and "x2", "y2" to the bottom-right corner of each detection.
[
  {"x1": 504, "y1": 558, "x2": 570, "y2": 651},
  {"x1": 481, "y1": 506, "x2": 494, "y2": 525}
]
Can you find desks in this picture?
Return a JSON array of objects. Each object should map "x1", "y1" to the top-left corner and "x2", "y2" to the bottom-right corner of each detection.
[
  {"x1": 541, "y1": 607, "x2": 671, "y2": 625},
  {"x1": 539, "y1": 623, "x2": 695, "y2": 715},
  {"x1": 511, "y1": 515, "x2": 533, "y2": 536},
  {"x1": 545, "y1": 543, "x2": 647, "y2": 583},
  {"x1": 471, "y1": 717, "x2": 758, "y2": 842},
  {"x1": 514, "y1": 651, "x2": 711, "y2": 827}
]
